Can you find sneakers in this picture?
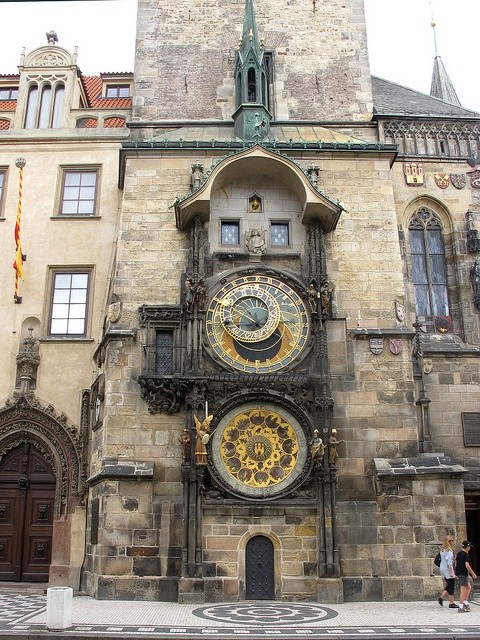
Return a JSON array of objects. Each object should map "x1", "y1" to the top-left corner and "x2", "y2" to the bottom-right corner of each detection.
[
  {"x1": 458, "y1": 607, "x2": 470, "y2": 613},
  {"x1": 464, "y1": 603, "x2": 469, "y2": 608}
]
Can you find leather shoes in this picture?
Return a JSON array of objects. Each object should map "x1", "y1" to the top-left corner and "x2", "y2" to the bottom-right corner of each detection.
[
  {"x1": 438, "y1": 598, "x2": 443, "y2": 606},
  {"x1": 449, "y1": 603, "x2": 458, "y2": 607}
]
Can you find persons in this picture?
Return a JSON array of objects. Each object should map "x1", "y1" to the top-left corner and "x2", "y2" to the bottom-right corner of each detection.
[
  {"x1": 455, "y1": 540, "x2": 477, "y2": 613},
  {"x1": 438, "y1": 536, "x2": 459, "y2": 608}
]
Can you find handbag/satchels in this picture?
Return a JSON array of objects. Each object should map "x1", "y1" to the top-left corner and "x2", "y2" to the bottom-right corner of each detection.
[{"x1": 434, "y1": 554, "x2": 441, "y2": 567}]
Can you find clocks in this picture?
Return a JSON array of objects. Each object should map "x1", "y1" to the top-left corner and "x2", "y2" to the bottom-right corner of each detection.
[{"x1": 201, "y1": 264, "x2": 318, "y2": 373}]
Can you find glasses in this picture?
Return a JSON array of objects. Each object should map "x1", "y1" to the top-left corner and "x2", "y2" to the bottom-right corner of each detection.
[{"x1": 450, "y1": 539, "x2": 453, "y2": 542}]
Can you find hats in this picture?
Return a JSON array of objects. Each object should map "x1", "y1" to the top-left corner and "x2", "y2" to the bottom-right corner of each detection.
[{"x1": 462, "y1": 541, "x2": 474, "y2": 548}]
